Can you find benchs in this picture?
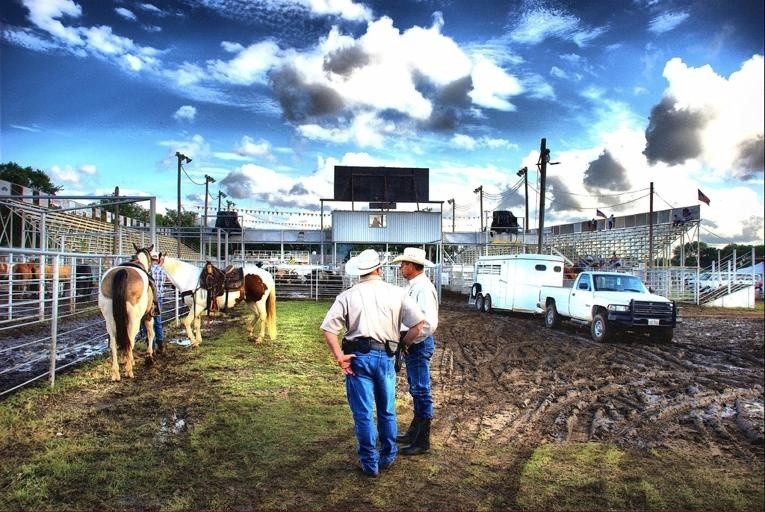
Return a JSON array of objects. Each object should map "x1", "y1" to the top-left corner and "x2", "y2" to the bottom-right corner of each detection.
[
  {"x1": 446, "y1": 221, "x2": 698, "y2": 269},
  {"x1": 7, "y1": 199, "x2": 208, "y2": 267}
]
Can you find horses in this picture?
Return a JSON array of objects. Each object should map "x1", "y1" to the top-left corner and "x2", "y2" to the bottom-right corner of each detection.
[
  {"x1": 97, "y1": 241, "x2": 157, "y2": 383},
  {"x1": 155, "y1": 250, "x2": 277, "y2": 348},
  {"x1": 0, "y1": 259, "x2": 94, "y2": 303}
]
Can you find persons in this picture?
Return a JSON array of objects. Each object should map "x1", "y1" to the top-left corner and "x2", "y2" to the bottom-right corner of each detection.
[
  {"x1": 142, "y1": 251, "x2": 166, "y2": 353},
  {"x1": 608, "y1": 214, "x2": 614, "y2": 231},
  {"x1": 390, "y1": 247, "x2": 441, "y2": 456},
  {"x1": 320, "y1": 247, "x2": 426, "y2": 476},
  {"x1": 591, "y1": 218, "x2": 596, "y2": 232},
  {"x1": 672, "y1": 214, "x2": 682, "y2": 226},
  {"x1": 566, "y1": 252, "x2": 623, "y2": 279}
]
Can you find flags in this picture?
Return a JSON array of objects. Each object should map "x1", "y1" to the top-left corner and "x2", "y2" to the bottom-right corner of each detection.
[
  {"x1": 597, "y1": 209, "x2": 609, "y2": 219},
  {"x1": 697, "y1": 189, "x2": 711, "y2": 205}
]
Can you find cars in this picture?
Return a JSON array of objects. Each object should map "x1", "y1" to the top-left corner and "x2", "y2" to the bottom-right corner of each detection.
[{"x1": 260, "y1": 259, "x2": 329, "y2": 285}]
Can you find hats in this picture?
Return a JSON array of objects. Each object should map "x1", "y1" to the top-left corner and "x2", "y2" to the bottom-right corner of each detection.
[
  {"x1": 389, "y1": 247, "x2": 437, "y2": 268},
  {"x1": 342, "y1": 249, "x2": 388, "y2": 278},
  {"x1": 150, "y1": 251, "x2": 159, "y2": 260}
]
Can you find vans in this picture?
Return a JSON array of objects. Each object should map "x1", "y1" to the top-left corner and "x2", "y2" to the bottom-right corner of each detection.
[{"x1": 688, "y1": 271, "x2": 761, "y2": 293}]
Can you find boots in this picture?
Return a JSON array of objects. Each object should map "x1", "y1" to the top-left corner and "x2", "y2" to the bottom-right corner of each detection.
[
  {"x1": 395, "y1": 410, "x2": 421, "y2": 444},
  {"x1": 156, "y1": 342, "x2": 166, "y2": 355},
  {"x1": 397, "y1": 418, "x2": 431, "y2": 456}
]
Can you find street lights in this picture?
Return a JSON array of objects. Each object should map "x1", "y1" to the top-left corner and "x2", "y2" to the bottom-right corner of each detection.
[
  {"x1": 174, "y1": 151, "x2": 236, "y2": 258},
  {"x1": 446, "y1": 166, "x2": 529, "y2": 254}
]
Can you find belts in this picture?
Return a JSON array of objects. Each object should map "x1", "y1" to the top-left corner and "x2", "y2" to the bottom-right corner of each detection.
[{"x1": 369, "y1": 342, "x2": 388, "y2": 354}]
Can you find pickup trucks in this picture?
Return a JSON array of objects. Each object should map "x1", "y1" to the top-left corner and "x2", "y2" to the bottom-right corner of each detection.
[{"x1": 536, "y1": 271, "x2": 678, "y2": 343}]
